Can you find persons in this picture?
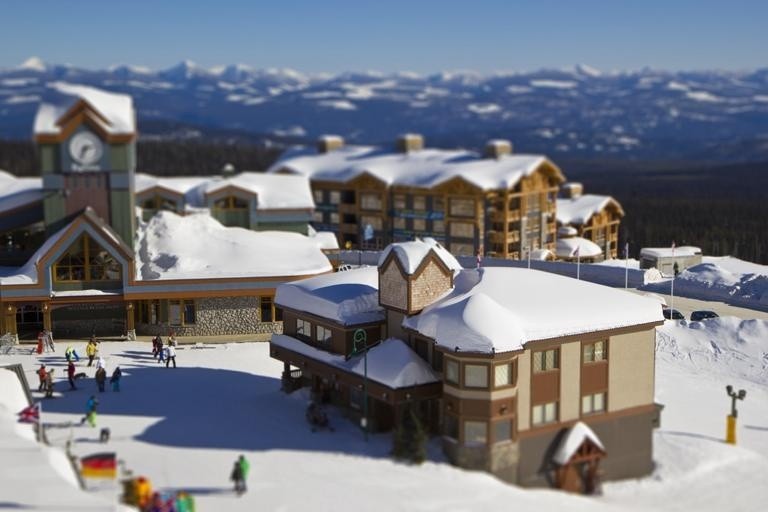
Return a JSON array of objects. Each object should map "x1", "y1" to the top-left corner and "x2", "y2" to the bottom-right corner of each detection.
[
  {"x1": 121, "y1": 469, "x2": 195, "y2": 512},
  {"x1": 152, "y1": 331, "x2": 178, "y2": 368},
  {"x1": 231, "y1": 456, "x2": 250, "y2": 493},
  {"x1": 100, "y1": 427, "x2": 111, "y2": 441},
  {"x1": 81, "y1": 395, "x2": 99, "y2": 427},
  {"x1": 36, "y1": 328, "x2": 121, "y2": 398}
]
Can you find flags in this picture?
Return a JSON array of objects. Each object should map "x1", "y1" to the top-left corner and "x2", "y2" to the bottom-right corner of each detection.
[
  {"x1": 81, "y1": 452, "x2": 117, "y2": 478},
  {"x1": 17, "y1": 403, "x2": 39, "y2": 423}
]
